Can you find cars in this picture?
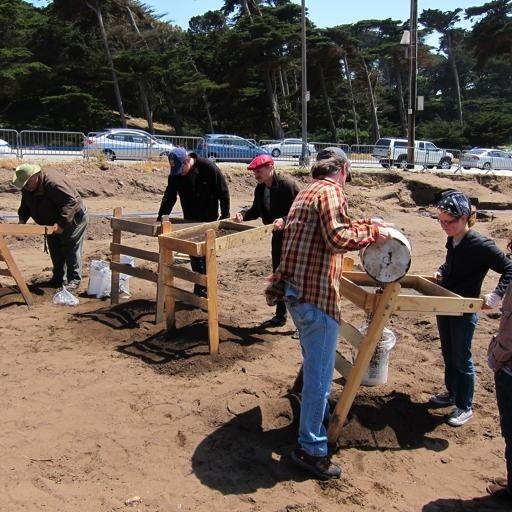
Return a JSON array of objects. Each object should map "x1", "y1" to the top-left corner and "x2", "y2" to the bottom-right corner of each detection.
[
  {"x1": 104, "y1": 128, "x2": 174, "y2": 147},
  {"x1": 260, "y1": 138, "x2": 317, "y2": 158},
  {"x1": 460, "y1": 147, "x2": 512, "y2": 171},
  {"x1": 83, "y1": 131, "x2": 177, "y2": 161},
  {"x1": 193, "y1": 134, "x2": 270, "y2": 164},
  {"x1": 0, "y1": 139, "x2": 13, "y2": 157}
]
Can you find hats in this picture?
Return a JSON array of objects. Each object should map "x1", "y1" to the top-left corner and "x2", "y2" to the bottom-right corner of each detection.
[
  {"x1": 316, "y1": 147, "x2": 353, "y2": 183},
  {"x1": 168, "y1": 147, "x2": 188, "y2": 176},
  {"x1": 12, "y1": 162, "x2": 41, "y2": 191},
  {"x1": 246, "y1": 155, "x2": 275, "y2": 170},
  {"x1": 438, "y1": 189, "x2": 472, "y2": 217}
]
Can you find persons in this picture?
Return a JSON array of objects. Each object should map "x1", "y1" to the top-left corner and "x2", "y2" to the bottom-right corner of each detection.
[
  {"x1": 235, "y1": 154, "x2": 302, "y2": 340},
  {"x1": 9, "y1": 163, "x2": 89, "y2": 289},
  {"x1": 263, "y1": 146, "x2": 390, "y2": 479},
  {"x1": 486, "y1": 237, "x2": 512, "y2": 502},
  {"x1": 431, "y1": 190, "x2": 512, "y2": 427},
  {"x1": 156, "y1": 148, "x2": 230, "y2": 313}
]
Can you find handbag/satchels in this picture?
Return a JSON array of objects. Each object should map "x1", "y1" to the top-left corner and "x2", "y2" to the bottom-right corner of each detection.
[{"x1": 87, "y1": 259, "x2": 130, "y2": 296}]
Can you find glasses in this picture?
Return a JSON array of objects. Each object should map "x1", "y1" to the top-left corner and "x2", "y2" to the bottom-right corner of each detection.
[{"x1": 437, "y1": 219, "x2": 460, "y2": 226}]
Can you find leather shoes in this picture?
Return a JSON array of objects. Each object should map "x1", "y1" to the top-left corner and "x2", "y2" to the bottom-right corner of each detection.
[
  {"x1": 44, "y1": 280, "x2": 63, "y2": 288},
  {"x1": 263, "y1": 317, "x2": 287, "y2": 327},
  {"x1": 64, "y1": 283, "x2": 79, "y2": 290}
]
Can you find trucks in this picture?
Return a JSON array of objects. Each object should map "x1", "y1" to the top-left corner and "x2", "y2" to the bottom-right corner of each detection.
[{"x1": 372, "y1": 138, "x2": 454, "y2": 170}]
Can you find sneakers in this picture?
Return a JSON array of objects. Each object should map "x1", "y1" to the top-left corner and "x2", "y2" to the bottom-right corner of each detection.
[
  {"x1": 447, "y1": 407, "x2": 474, "y2": 425},
  {"x1": 291, "y1": 445, "x2": 342, "y2": 479},
  {"x1": 429, "y1": 390, "x2": 456, "y2": 405}
]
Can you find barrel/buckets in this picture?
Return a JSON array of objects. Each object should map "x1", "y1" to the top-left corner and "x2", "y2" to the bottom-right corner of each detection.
[
  {"x1": 349, "y1": 325, "x2": 397, "y2": 388},
  {"x1": 359, "y1": 227, "x2": 413, "y2": 285},
  {"x1": 86, "y1": 259, "x2": 111, "y2": 299},
  {"x1": 341, "y1": 256, "x2": 355, "y2": 272}
]
